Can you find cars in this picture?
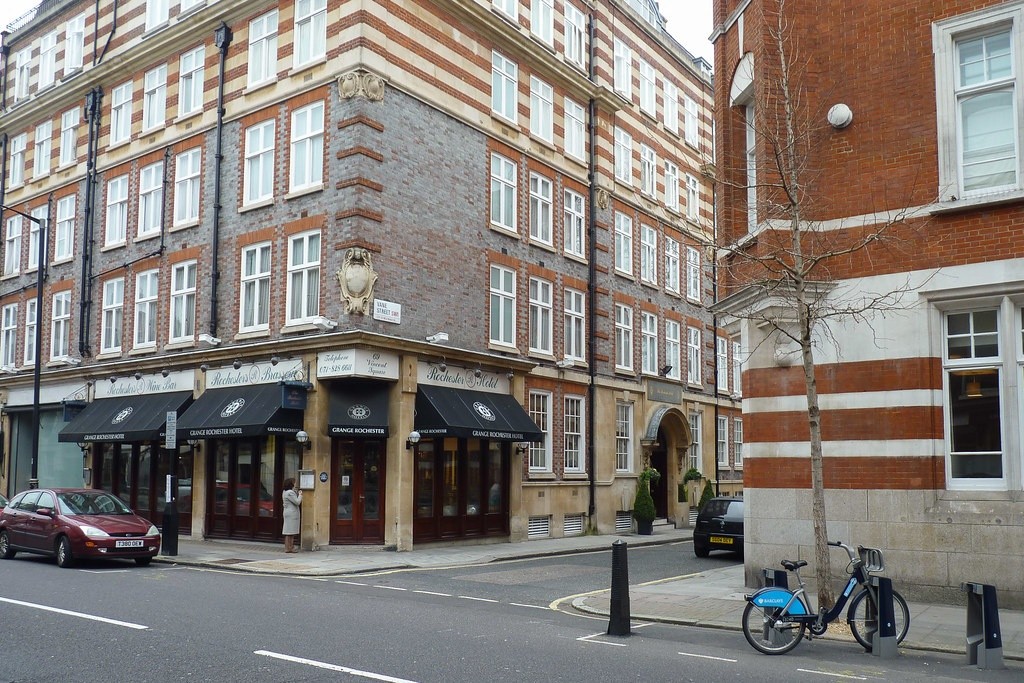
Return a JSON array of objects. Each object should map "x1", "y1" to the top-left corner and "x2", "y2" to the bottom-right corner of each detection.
[
  {"x1": 177, "y1": 477, "x2": 229, "y2": 499},
  {"x1": 0, "y1": 487, "x2": 162, "y2": 569},
  {"x1": 692, "y1": 495, "x2": 745, "y2": 558},
  {"x1": 0, "y1": 492, "x2": 10, "y2": 523},
  {"x1": 176, "y1": 483, "x2": 275, "y2": 517}
]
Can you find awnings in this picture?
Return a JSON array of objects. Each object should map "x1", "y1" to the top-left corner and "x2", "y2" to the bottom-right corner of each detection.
[
  {"x1": 414, "y1": 384, "x2": 543, "y2": 442},
  {"x1": 328, "y1": 383, "x2": 390, "y2": 438},
  {"x1": 176, "y1": 383, "x2": 303, "y2": 440},
  {"x1": 57, "y1": 391, "x2": 195, "y2": 442}
]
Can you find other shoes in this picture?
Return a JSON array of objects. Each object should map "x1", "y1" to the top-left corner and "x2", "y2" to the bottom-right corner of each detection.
[{"x1": 285, "y1": 550, "x2": 298, "y2": 553}]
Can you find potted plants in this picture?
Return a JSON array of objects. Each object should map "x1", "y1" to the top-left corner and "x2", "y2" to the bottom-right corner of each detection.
[{"x1": 633, "y1": 478, "x2": 657, "y2": 535}]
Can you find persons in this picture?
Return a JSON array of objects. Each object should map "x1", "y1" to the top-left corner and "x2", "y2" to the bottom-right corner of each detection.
[{"x1": 282, "y1": 478, "x2": 303, "y2": 553}]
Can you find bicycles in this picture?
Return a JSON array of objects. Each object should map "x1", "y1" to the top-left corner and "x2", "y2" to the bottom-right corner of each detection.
[{"x1": 741, "y1": 539, "x2": 911, "y2": 656}]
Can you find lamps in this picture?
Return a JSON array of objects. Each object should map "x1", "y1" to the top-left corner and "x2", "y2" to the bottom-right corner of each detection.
[
  {"x1": 516, "y1": 442, "x2": 531, "y2": 455},
  {"x1": 78, "y1": 443, "x2": 91, "y2": 454},
  {"x1": 199, "y1": 333, "x2": 221, "y2": 345},
  {"x1": 312, "y1": 316, "x2": 338, "y2": 331},
  {"x1": 730, "y1": 393, "x2": 742, "y2": 400},
  {"x1": 162, "y1": 362, "x2": 182, "y2": 378},
  {"x1": 188, "y1": 440, "x2": 201, "y2": 452},
  {"x1": 427, "y1": 355, "x2": 447, "y2": 373},
  {"x1": 406, "y1": 431, "x2": 421, "y2": 449},
  {"x1": 967, "y1": 375, "x2": 983, "y2": 397},
  {"x1": 556, "y1": 358, "x2": 575, "y2": 368},
  {"x1": 86, "y1": 372, "x2": 106, "y2": 387},
  {"x1": 61, "y1": 354, "x2": 81, "y2": 366},
  {"x1": 296, "y1": 430, "x2": 312, "y2": 450},
  {"x1": 271, "y1": 347, "x2": 291, "y2": 366},
  {"x1": 496, "y1": 365, "x2": 514, "y2": 381},
  {"x1": 660, "y1": 365, "x2": 672, "y2": 374},
  {"x1": 1, "y1": 365, "x2": 20, "y2": 374},
  {"x1": 135, "y1": 364, "x2": 156, "y2": 380},
  {"x1": 200, "y1": 357, "x2": 222, "y2": 373},
  {"x1": 465, "y1": 361, "x2": 482, "y2": 378},
  {"x1": 426, "y1": 332, "x2": 449, "y2": 344},
  {"x1": 233, "y1": 352, "x2": 255, "y2": 371},
  {"x1": 110, "y1": 369, "x2": 131, "y2": 384}
]
